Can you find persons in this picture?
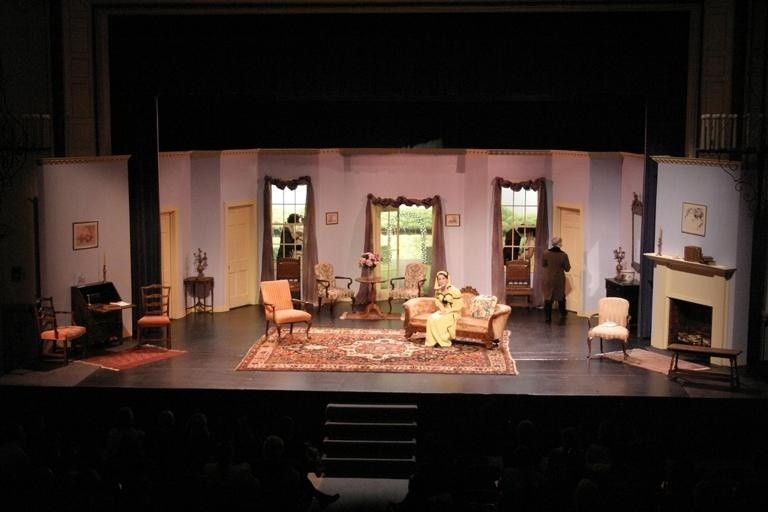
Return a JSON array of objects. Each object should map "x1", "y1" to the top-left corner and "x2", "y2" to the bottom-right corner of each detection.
[
  {"x1": 541, "y1": 237, "x2": 571, "y2": 327},
  {"x1": 277, "y1": 215, "x2": 304, "y2": 259},
  {"x1": 425, "y1": 271, "x2": 462, "y2": 348},
  {"x1": 523, "y1": 231, "x2": 536, "y2": 290}
]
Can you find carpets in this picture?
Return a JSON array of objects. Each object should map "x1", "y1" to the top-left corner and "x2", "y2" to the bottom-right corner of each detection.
[
  {"x1": 591, "y1": 348, "x2": 711, "y2": 376},
  {"x1": 70, "y1": 337, "x2": 189, "y2": 374},
  {"x1": 339, "y1": 312, "x2": 403, "y2": 321},
  {"x1": 233, "y1": 324, "x2": 519, "y2": 376}
]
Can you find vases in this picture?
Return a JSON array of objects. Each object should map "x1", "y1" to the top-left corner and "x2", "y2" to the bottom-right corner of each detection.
[
  {"x1": 615, "y1": 263, "x2": 623, "y2": 281},
  {"x1": 196, "y1": 263, "x2": 204, "y2": 277}
]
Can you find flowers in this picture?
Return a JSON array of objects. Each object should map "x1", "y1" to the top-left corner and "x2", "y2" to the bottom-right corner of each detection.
[{"x1": 358, "y1": 252, "x2": 382, "y2": 267}]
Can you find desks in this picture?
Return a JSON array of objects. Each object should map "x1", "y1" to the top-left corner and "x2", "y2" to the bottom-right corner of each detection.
[{"x1": 183, "y1": 276, "x2": 214, "y2": 319}]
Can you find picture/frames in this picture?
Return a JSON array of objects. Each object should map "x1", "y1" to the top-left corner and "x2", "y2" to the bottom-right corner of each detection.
[
  {"x1": 72, "y1": 221, "x2": 99, "y2": 251},
  {"x1": 680, "y1": 201, "x2": 707, "y2": 238},
  {"x1": 445, "y1": 214, "x2": 460, "y2": 228},
  {"x1": 327, "y1": 212, "x2": 338, "y2": 225}
]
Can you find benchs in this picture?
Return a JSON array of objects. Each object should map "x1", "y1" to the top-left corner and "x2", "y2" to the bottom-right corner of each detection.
[{"x1": 668, "y1": 343, "x2": 743, "y2": 392}]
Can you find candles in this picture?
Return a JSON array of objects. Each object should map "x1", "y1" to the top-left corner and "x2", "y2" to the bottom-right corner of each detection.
[{"x1": 103, "y1": 251, "x2": 108, "y2": 265}]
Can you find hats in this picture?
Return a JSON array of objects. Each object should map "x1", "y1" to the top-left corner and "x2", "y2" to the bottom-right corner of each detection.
[{"x1": 287, "y1": 214, "x2": 300, "y2": 223}]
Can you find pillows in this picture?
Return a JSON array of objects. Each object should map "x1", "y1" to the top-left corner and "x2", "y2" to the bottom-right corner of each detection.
[{"x1": 469, "y1": 295, "x2": 498, "y2": 319}]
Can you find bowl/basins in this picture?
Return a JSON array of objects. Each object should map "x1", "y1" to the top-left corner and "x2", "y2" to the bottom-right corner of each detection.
[{"x1": 621, "y1": 271, "x2": 635, "y2": 285}]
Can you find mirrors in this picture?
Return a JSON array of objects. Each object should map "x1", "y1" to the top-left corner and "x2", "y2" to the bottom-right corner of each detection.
[{"x1": 631, "y1": 192, "x2": 643, "y2": 275}]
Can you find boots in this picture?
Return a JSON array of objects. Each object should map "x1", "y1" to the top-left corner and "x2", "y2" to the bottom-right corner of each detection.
[
  {"x1": 559, "y1": 300, "x2": 566, "y2": 315},
  {"x1": 545, "y1": 303, "x2": 551, "y2": 320}
]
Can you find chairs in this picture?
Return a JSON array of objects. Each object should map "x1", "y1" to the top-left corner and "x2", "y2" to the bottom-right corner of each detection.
[
  {"x1": 277, "y1": 254, "x2": 301, "y2": 309},
  {"x1": 31, "y1": 284, "x2": 172, "y2": 366},
  {"x1": 505, "y1": 260, "x2": 534, "y2": 312},
  {"x1": 586, "y1": 297, "x2": 631, "y2": 360},
  {"x1": 256, "y1": 262, "x2": 427, "y2": 342}
]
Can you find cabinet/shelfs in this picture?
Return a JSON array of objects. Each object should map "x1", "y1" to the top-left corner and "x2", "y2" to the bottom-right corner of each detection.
[{"x1": 606, "y1": 275, "x2": 640, "y2": 347}]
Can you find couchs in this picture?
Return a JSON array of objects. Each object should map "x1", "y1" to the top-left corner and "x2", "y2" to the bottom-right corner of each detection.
[{"x1": 405, "y1": 286, "x2": 513, "y2": 350}]
